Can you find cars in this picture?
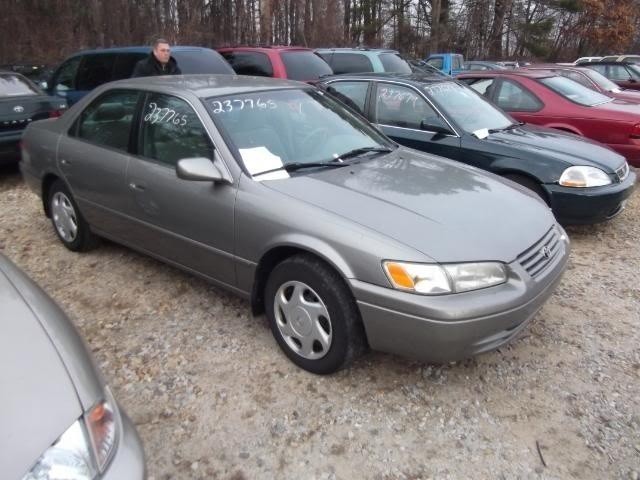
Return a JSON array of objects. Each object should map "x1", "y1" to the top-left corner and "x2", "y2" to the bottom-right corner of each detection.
[
  {"x1": 215, "y1": 47, "x2": 333, "y2": 82},
  {"x1": 409, "y1": 58, "x2": 451, "y2": 78},
  {"x1": 0, "y1": 248, "x2": 149, "y2": 480},
  {"x1": 525, "y1": 63, "x2": 639, "y2": 103},
  {"x1": 19, "y1": 74, "x2": 572, "y2": 372},
  {"x1": 455, "y1": 73, "x2": 640, "y2": 166},
  {"x1": 463, "y1": 62, "x2": 506, "y2": 70},
  {"x1": 308, "y1": 74, "x2": 636, "y2": 222},
  {"x1": 1, "y1": 62, "x2": 69, "y2": 166},
  {"x1": 576, "y1": 55, "x2": 639, "y2": 89},
  {"x1": 313, "y1": 48, "x2": 413, "y2": 75},
  {"x1": 497, "y1": 62, "x2": 520, "y2": 69}
]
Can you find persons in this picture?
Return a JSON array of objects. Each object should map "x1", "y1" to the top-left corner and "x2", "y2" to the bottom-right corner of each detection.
[{"x1": 124, "y1": 38, "x2": 182, "y2": 117}]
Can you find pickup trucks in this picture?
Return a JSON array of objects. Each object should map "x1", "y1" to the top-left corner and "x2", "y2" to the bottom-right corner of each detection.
[{"x1": 424, "y1": 55, "x2": 482, "y2": 73}]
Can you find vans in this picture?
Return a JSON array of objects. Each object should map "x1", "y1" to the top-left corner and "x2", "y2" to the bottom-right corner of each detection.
[{"x1": 44, "y1": 48, "x2": 234, "y2": 115}]
